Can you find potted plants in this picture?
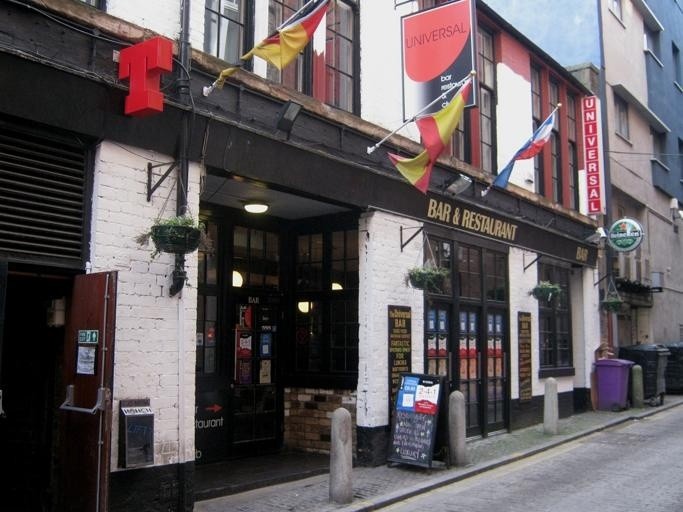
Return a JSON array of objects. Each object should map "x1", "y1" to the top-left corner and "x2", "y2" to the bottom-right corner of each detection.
[
  {"x1": 140, "y1": 216, "x2": 210, "y2": 263},
  {"x1": 530, "y1": 281, "x2": 561, "y2": 303},
  {"x1": 602, "y1": 296, "x2": 624, "y2": 312},
  {"x1": 406, "y1": 264, "x2": 450, "y2": 291}
]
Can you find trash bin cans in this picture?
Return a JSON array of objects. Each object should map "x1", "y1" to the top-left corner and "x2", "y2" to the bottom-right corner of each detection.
[{"x1": 593, "y1": 342, "x2": 683, "y2": 412}]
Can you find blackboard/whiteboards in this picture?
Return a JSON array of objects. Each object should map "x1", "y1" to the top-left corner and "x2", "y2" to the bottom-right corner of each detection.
[
  {"x1": 518, "y1": 312, "x2": 532, "y2": 403},
  {"x1": 386, "y1": 305, "x2": 442, "y2": 469}
]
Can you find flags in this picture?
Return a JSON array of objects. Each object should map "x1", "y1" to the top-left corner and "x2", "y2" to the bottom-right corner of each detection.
[
  {"x1": 209, "y1": 0, "x2": 335, "y2": 91},
  {"x1": 379, "y1": 79, "x2": 472, "y2": 197},
  {"x1": 488, "y1": 112, "x2": 558, "y2": 189}
]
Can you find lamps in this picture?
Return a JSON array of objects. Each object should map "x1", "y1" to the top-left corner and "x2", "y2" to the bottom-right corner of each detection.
[
  {"x1": 275, "y1": 100, "x2": 303, "y2": 137},
  {"x1": 438, "y1": 174, "x2": 472, "y2": 194}
]
